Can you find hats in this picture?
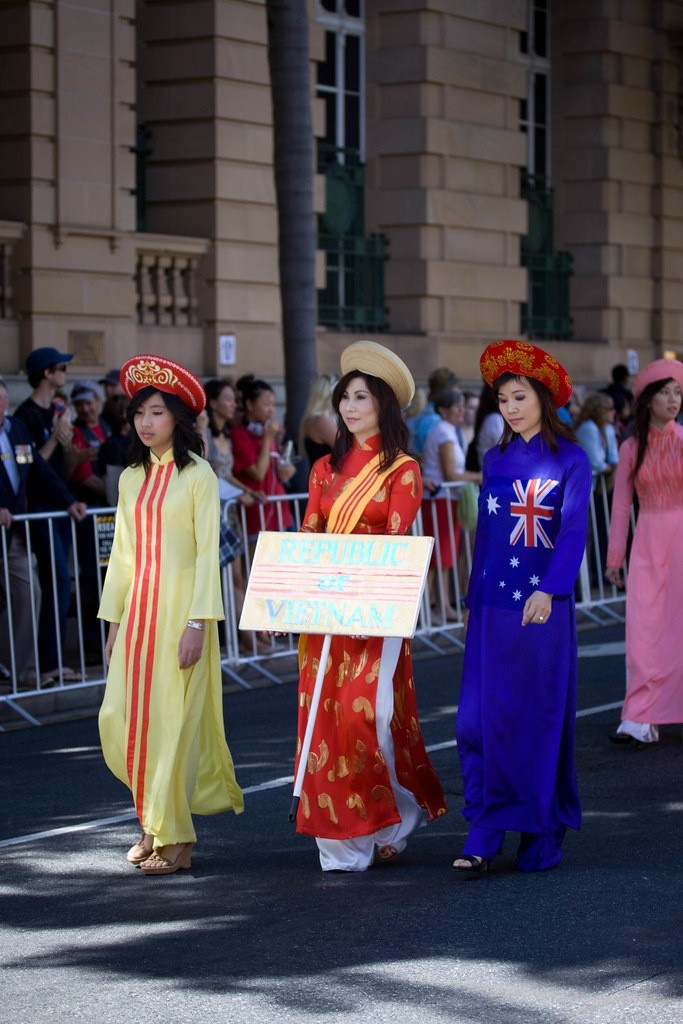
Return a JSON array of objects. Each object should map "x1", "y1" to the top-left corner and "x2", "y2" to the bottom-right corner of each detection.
[
  {"x1": 100, "y1": 370, "x2": 121, "y2": 386},
  {"x1": 341, "y1": 343, "x2": 416, "y2": 413},
  {"x1": 632, "y1": 358, "x2": 683, "y2": 400},
  {"x1": 71, "y1": 379, "x2": 98, "y2": 403},
  {"x1": 24, "y1": 347, "x2": 74, "y2": 379},
  {"x1": 120, "y1": 354, "x2": 206, "y2": 415},
  {"x1": 481, "y1": 340, "x2": 573, "y2": 416},
  {"x1": 427, "y1": 368, "x2": 462, "y2": 400}
]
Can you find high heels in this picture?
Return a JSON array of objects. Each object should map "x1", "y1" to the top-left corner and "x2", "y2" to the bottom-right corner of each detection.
[
  {"x1": 127, "y1": 834, "x2": 157, "y2": 866},
  {"x1": 452, "y1": 852, "x2": 487, "y2": 873},
  {"x1": 140, "y1": 839, "x2": 193, "y2": 874}
]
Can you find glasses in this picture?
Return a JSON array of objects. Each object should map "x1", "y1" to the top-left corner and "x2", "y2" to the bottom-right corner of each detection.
[{"x1": 45, "y1": 364, "x2": 67, "y2": 373}]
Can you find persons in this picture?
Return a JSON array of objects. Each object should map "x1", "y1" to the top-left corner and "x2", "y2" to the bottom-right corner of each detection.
[
  {"x1": 0, "y1": 346, "x2": 634, "y2": 695},
  {"x1": 99, "y1": 353, "x2": 246, "y2": 875},
  {"x1": 259, "y1": 339, "x2": 447, "y2": 872},
  {"x1": 449, "y1": 340, "x2": 593, "y2": 878},
  {"x1": 604, "y1": 358, "x2": 683, "y2": 743}
]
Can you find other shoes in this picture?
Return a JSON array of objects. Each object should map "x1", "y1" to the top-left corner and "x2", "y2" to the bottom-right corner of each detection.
[
  {"x1": 610, "y1": 731, "x2": 632, "y2": 745},
  {"x1": 377, "y1": 837, "x2": 405, "y2": 862},
  {"x1": 10, "y1": 671, "x2": 55, "y2": 694}
]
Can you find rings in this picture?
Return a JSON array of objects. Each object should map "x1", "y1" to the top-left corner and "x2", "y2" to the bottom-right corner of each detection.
[{"x1": 540, "y1": 617, "x2": 543, "y2": 620}]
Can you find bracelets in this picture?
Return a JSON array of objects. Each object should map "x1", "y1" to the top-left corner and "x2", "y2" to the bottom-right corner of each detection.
[{"x1": 188, "y1": 621, "x2": 205, "y2": 630}]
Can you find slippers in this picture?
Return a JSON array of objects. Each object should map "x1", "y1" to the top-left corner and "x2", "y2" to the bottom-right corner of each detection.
[{"x1": 47, "y1": 668, "x2": 85, "y2": 684}]
